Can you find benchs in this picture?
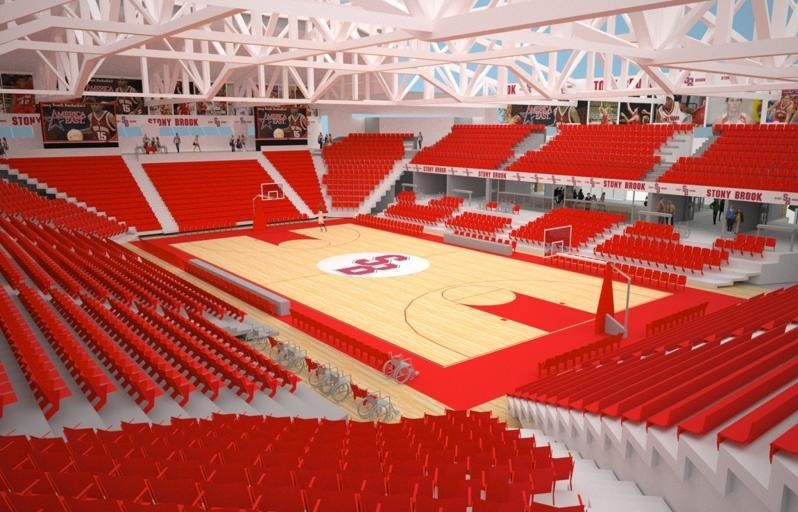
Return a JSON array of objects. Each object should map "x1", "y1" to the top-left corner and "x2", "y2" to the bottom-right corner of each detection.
[{"x1": 508, "y1": 285, "x2": 798, "y2": 473}]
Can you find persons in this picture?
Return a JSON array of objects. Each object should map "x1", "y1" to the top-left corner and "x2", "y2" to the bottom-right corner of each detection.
[
  {"x1": 598, "y1": 101, "x2": 609, "y2": 124},
  {"x1": 143, "y1": 135, "x2": 162, "y2": 154},
  {"x1": 417, "y1": 132, "x2": 423, "y2": 149},
  {"x1": 284, "y1": 109, "x2": 307, "y2": 138},
  {"x1": 100, "y1": 79, "x2": 143, "y2": 115},
  {"x1": 767, "y1": 93, "x2": 796, "y2": 124},
  {"x1": 654, "y1": 97, "x2": 692, "y2": 123},
  {"x1": 10, "y1": 75, "x2": 36, "y2": 113},
  {"x1": 0, "y1": 137, "x2": 8, "y2": 158},
  {"x1": 554, "y1": 186, "x2": 606, "y2": 210},
  {"x1": 621, "y1": 103, "x2": 650, "y2": 123},
  {"x1": 193, "y1": 135, "x2": 201, "y2": 151},
  {"x1": 173, "y1": 133, "x2": 181, "y2": 152},
  {"x1": 317, "y1": 209, "x2": 327, "y2": 233},
  {"x1": 318, "y1": 133, "x2": 333, "y2": 148},
  {"x1": 231, "y1": 134, "x2": 246, "y2": 152},
  {"x1": 80, "y1": 103, "x2": 117, "y2": 141},
  {"x1": 713, "y1": 97, "x2": 753, "y2": 125},
  {"x1": 549, "y1": 105, "x2": 580, "y2": 127},
  {"x1": 510, "y1": 113, "x2": 523, "y2": 125},
  {"x1": 658, "y1": 196, "x2": 745, "y2": 232}
]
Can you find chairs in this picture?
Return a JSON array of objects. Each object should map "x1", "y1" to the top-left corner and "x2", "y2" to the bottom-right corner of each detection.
[
  {"x1": 592, "y1": 219, "x2": 776, "y2": 294},
  {"x1": 1, "y1": 284, "x2": 586, "y2": 512}
]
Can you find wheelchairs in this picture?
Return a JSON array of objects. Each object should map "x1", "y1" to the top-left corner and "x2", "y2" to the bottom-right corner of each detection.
[
  {"x1": 244, "y1": 324, "x2": 350, "y2": 402},
  {"x1": 383, "y1": 351, "x2": 421, "y2": 384},
  {"x1": 357, "y1": 389, "x2": 399, "y2": 423}
]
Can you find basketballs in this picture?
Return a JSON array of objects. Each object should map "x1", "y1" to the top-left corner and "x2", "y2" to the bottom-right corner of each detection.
[
  {"x1": 274, "y1": 129, "x2": 284, "y2": 138},
  {"x1": 67, "y1": 129, "x2": 83, "y2": 142}
]
